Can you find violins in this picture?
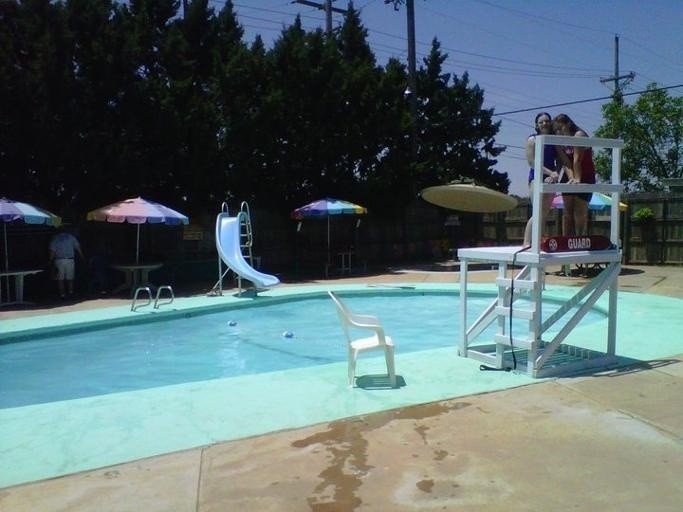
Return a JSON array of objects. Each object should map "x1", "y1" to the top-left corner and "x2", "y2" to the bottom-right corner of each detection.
[{"x1": 215, "y1": 211, "x2": 280, "y2": 287}]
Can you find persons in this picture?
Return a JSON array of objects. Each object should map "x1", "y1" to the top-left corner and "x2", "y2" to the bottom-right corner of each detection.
[
  {"x1": 544, "y1": 114, "x2": 595, "y2": 237},
  {"x1": 47, "y1": 227, "x2": 86, "y2": 299},
  {"x1": 523, "y1": 112, "x2": 574, "y2": 251}
]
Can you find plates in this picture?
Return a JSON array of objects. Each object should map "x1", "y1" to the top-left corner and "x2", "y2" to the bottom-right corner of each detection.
[{"x1": 480, "y1": 196, "x2": 683, "y2": 265}]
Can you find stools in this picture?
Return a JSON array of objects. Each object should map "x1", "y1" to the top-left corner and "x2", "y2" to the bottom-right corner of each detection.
[{"x1": 56, "y1": 256, "x2": 74, "y2": 260}]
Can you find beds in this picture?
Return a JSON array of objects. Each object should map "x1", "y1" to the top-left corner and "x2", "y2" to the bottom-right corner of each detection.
[
  {"x1": 0, "y1": 195, "x2": 63, "y2": 303},
  {"x1": 86, "y1": 195, "x2": 189, "y2": 288},
  {"x1": 288, "y1": 196, "x2": 368, "y2": 264},
  {"x1": 551, "y1": 192, "x2": 629, "y2": 211}
]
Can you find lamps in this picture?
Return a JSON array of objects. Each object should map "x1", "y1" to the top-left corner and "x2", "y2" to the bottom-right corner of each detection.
[
  {"x1": 325, "y1": 252, "x2": 351, "y2": 278},
  {"x1": 328, "y1": 290, "x2": 398, "y2": 387}
]
